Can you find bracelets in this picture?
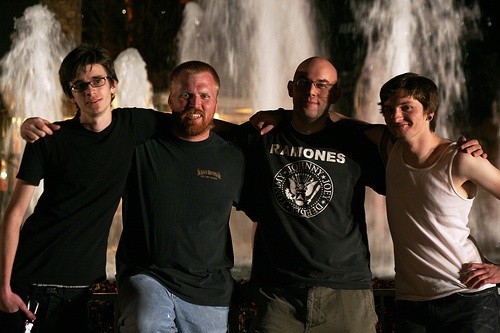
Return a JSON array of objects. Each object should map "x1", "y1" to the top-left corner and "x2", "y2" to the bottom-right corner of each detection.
[{"x1": 279, "y1": 107, "x2": 286, "y2": 124}]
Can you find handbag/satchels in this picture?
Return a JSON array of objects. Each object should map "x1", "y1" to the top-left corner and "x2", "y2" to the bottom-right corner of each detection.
[
  {"x1": 230, "y1": 294, "x2": 259, "y2": 332},
  {"x1": 83, "y1": 279, "x2": 119, "y2": 319}
]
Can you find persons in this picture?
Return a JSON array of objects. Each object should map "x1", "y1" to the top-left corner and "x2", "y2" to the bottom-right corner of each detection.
[
  {"x1": 0, "y1": 42, "x2": 150, "y2": 333},
  {"x1": 19, "y1": 59, "x2": 250, "y2": 333},
  {"x1": 250, "y1": 71, "x2": 500, "y2": 332},
  {"x1": 237, "y1": 53, "x2": 488, "y2": 331}
]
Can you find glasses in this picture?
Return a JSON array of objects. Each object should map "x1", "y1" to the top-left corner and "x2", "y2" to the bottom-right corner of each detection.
[
  {"x1": 293, "y1": 79, "x2": 335, "y2": 92},
  {"x1": 69, "y1": 75, "x2": 113, "y2": 93}
]
